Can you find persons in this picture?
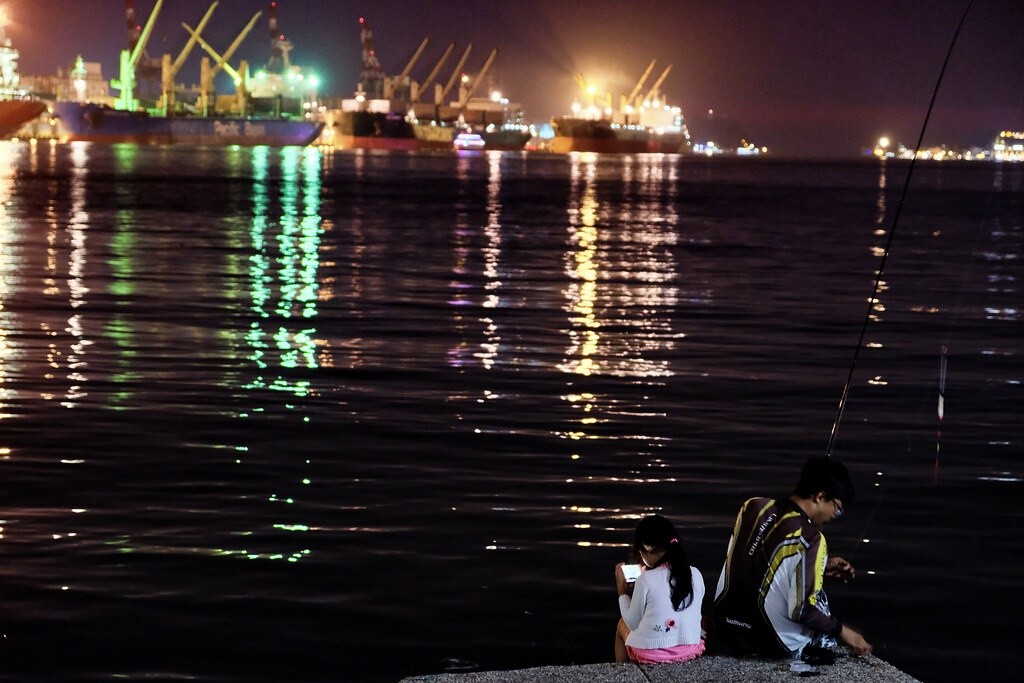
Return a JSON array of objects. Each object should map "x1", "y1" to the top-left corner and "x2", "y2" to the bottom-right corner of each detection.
[
  {"x1": 713, "y1": 471, "x2": 872, "y2": 659},
  {"x1": 612, "y1": 514, "x2": 706, "y2": 665}
]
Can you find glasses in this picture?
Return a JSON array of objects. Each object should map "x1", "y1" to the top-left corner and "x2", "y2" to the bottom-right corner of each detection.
[{"x1": 831, "y1": 498, "x2": 845, "y2": 516}]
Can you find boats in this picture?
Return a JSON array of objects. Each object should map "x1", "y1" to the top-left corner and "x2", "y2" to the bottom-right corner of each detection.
[
  {"x1": 0, "y1": 89, "x2": 48, "y2": 135},
  {"x1": 48, "y1": 99, "x2": 328, "y2": 149}
]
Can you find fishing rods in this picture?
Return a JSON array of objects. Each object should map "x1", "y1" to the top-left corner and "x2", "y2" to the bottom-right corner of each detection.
[{"x1": 821, "y1": 0, "x2": 977, "y2": 458}]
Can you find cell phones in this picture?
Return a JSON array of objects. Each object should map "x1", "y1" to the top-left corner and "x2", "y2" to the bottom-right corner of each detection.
[{"x1": 620, "y1": 563, "x2": 643, "y2": 584}]
